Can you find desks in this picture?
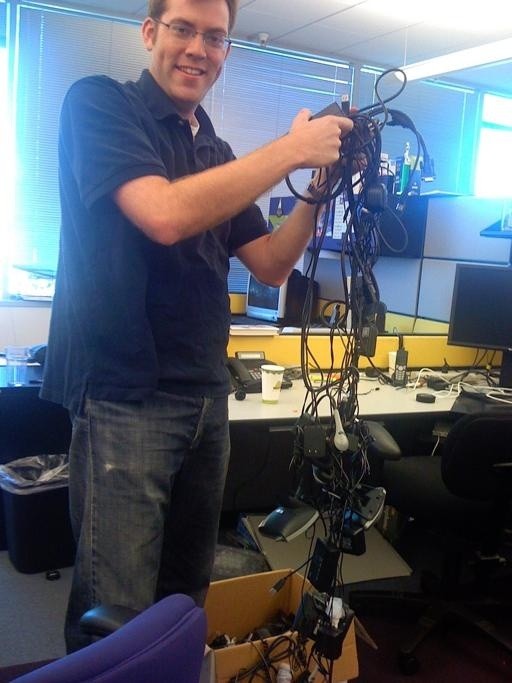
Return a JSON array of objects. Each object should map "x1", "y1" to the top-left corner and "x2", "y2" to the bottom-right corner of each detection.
[{"x1": 220, "y1": 368, "x2": 512, "y2": 542}]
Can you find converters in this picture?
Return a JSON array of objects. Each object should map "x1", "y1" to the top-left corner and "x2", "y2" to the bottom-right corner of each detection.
[{"x1": 259, "y1": 97, "x2": 396, "y2": 662}]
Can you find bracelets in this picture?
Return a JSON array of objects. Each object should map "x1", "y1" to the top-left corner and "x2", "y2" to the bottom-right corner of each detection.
[{"x1": 307, "y1": 184, "x2": 323, "y2": 197}]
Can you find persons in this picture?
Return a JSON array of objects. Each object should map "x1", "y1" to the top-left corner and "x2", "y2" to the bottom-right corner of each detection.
[{"x1": 39, "y1": 0, "x2": 367, "y2": 654}]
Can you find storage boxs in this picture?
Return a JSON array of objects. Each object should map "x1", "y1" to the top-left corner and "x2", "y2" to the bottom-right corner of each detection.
[{"x1": 206, "y1": 571, "x2": 376, "y2": 683}]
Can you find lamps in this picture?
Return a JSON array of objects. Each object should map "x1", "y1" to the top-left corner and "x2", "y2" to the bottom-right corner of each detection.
[{"x1": 394, "y1": 28, "x2": 512, "y2": 82}]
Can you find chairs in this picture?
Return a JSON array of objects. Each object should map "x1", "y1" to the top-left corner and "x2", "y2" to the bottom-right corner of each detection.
[
  {"x1": 384, "y1": 406, "x2": 512, "y2": 675},
  {"x1": 0, "y1": 592, "x2": 205, "y2": 683}
]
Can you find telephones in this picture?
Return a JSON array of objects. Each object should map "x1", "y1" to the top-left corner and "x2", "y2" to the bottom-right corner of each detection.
[
  {"x1": 391, "y1": 334, "x2": 408, "y2": 387},
  {"x1": 226, "y1": 351, "x2": 293, "y2": 393}
]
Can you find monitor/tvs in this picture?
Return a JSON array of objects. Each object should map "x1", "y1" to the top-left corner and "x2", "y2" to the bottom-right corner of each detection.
[
  {"x1": 447, "y1": 261, "x2": 512, "y2": 353},
  {"x1": 245, "y1": 268, "x2": 318, "y2": 327}
]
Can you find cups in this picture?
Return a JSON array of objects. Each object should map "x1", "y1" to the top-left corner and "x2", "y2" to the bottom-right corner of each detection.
[
  {"x1": 2, "y1": 344, "x2": 33, "y2": 387},
  {"x1": 260, "y1": 364, "x2": 286, "y2": 405},
  {"x1": 389, "y1": 351, "x2": 397, "y2": 377}
]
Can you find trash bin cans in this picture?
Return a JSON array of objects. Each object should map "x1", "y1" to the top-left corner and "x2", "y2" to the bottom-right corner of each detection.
[{"x1": 0, "y1": 454, "x2": 76, "y2": 574}]
